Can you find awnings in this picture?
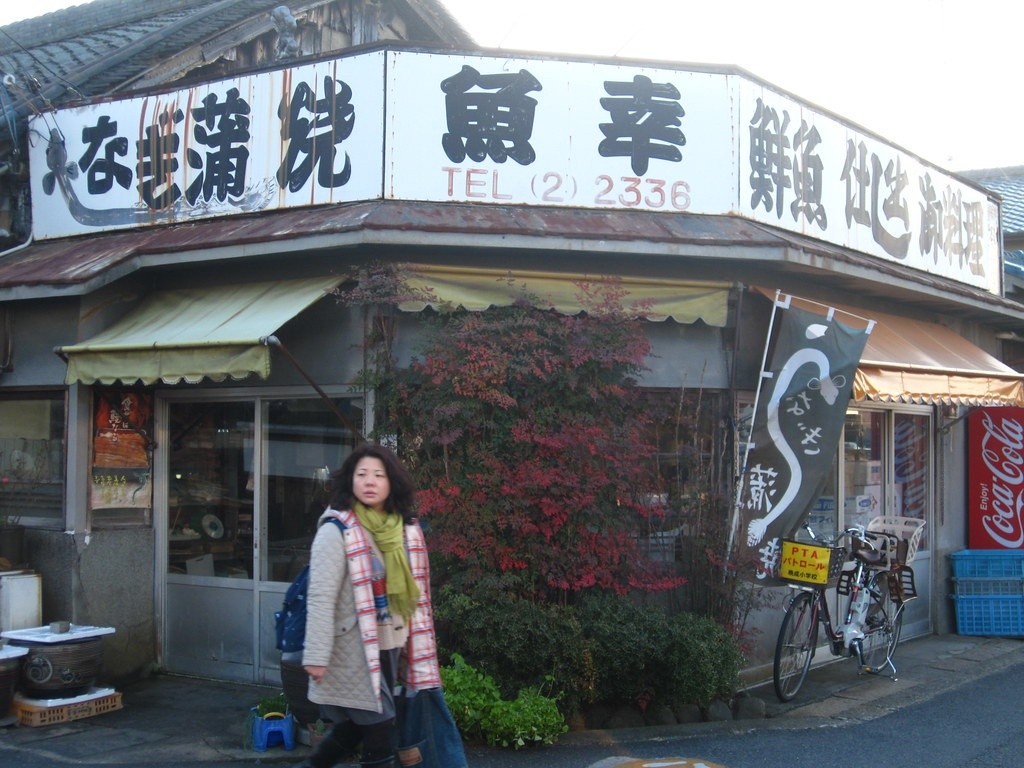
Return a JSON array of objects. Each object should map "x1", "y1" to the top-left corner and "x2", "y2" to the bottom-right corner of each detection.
[
  {"x1": 744, "y1": 283, "x2": 1023, "y2": 409},
  {"x1": 385, "y1": 262, "x2": 735, "y2": 328},
  {"x1": 52, "y1": 273, "x2": 373, "y2": 449}
]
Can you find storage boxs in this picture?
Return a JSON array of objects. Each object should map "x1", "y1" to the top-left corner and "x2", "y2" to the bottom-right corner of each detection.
[
  {"x1": 949, "y1": 550, "x2": 1024, "y2": 636},
  {"x1": 802, "y1": 461, "x2": 903, "y2": 544}
]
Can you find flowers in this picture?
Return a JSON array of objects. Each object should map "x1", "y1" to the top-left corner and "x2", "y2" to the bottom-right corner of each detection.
[{"x1": 0, "y1": 447, "x2": 46, "y2": 529}]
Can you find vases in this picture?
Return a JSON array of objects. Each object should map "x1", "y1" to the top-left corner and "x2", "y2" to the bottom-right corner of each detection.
[{"x1": 0, "y1": 526, "x2": 24, "y2": 553}]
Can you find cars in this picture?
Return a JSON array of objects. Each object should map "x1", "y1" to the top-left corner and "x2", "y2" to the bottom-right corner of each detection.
[{"x1": 845, "y1": 442, "x2": 871, "y2": 459}]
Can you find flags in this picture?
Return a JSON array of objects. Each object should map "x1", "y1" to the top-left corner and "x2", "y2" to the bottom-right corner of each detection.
[{"x1": 725, "y1": 305, "x2": 871, "y2": 576}]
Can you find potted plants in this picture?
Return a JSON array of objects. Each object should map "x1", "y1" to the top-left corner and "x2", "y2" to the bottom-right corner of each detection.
[
  {"x1": 307, "y1": 719, "x2": 335, "y2": 748},
  {"x1": 243, "y1": 695, "x2": 294, "y2": 753},
  {"x1": 439, "y1": 653, "x2": 568, "y2": 753}
]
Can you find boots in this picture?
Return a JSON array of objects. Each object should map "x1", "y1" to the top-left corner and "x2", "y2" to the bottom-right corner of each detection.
[
  {"x1": 295, "y1": 732, "x2": 349, "y2": 768},
  {"x1": 360, "y1": 756, "x2": 395, "y2": 768}
]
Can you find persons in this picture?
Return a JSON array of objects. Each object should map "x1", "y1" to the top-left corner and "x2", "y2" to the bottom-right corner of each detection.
[{"x1": 301, "y1": 442, "x2": 442, "y2": 768}]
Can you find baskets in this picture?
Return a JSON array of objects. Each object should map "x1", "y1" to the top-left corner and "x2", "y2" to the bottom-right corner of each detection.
[{"x1": 779, "y1": 538, "x2": 848, "y2": 590}]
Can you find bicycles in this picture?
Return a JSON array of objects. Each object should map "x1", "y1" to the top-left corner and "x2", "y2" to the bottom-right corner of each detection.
[{"x1": 774, "y1": 520, "x2": 905, "y2": 704}]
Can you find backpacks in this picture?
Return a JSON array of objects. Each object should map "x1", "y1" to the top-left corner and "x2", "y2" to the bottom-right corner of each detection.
[{"x1": 274, "y1": 518, "x2": 349, "y2": 652}]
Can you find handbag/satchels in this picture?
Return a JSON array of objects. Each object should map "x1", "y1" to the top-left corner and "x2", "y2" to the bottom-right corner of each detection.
[{"x1": 393, "y1": 686, "x2": 468, "y2": 768}]
